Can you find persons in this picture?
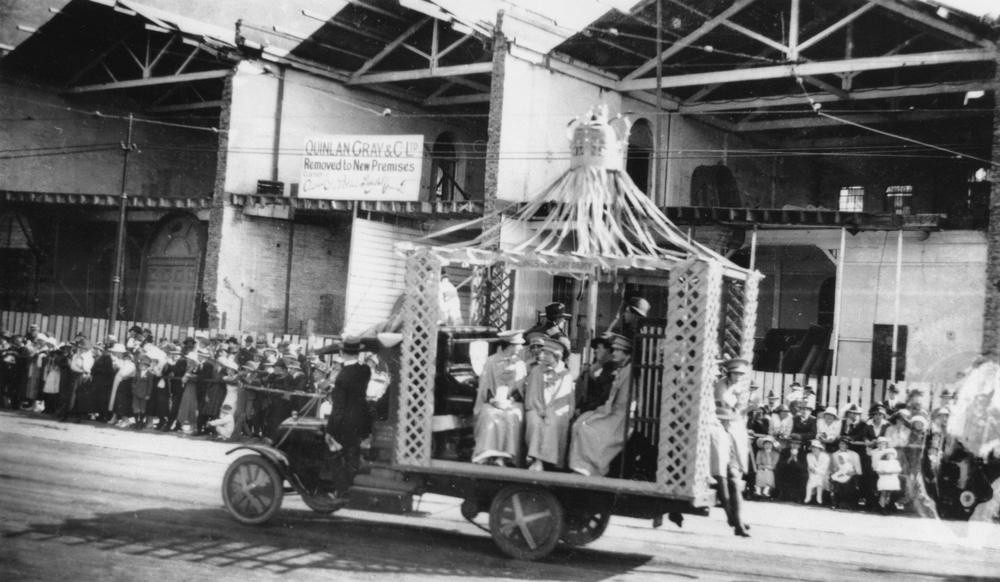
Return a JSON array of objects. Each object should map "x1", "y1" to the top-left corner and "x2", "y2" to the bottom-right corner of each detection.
[
  {"x1": 524, "y1": 301, "x2": 574, "y2": 354},
  {"x1": 744, "y1": 380, "x2": 1000, "y2": 524},
  {"x1": 710, "y1": 403, "x2": 752, "y2": 540},
  {"x1": 715, "y1": 357, "x2": 753, "y2": 476},
  {"x1": 324, "y1": 335, "x2": 372, "y2": 497},
  {"x1": 471, "y1": 328, "x2": 527, "y2": 466},
  {"x1": 524, "y1": 339, "x2": 578, "y2": 470},
  {"x1": 621, "y1": 294, "x2": 652, "y2": 340},
  {"x1": 564, "y1": 331, "x2": 617, "y2": 467},
  {"x1": 439, "y1": 265, "x2": 463, "y2": 327},
  {"x1": 0, "y1": 325, "x2": 329, "y2": 443},
  {"x1": 568, "y1": 335, "x2": 635, "y2": 475}
]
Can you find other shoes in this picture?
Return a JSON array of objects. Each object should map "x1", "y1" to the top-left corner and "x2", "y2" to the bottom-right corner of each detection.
[{"x1": 727, "y1": 518, "x2": 751, "y2": 537}]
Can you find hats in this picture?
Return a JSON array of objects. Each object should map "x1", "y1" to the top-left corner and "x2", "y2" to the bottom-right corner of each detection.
[
  {"x1": 220, "y1": 404, "x2": 232, "y2": 414},
  {"x1": 590, "y1": 331, "x2": 617, "y2": 350},
  {"x1": 624, "y1": 296, "x2": 651, "y2": 318},
  {"x1": 0, "y1": 323, "x2": 334, "y2": 378},
  {"x1": 609, "y1": 333, "x2": 633, "y2": 353},
  {"x1": 539, "y1": 302, "x2": 573, "y2": 318},
  {"x1": 750, "y1": 378, "x2": 958, "y2": 461},
  {"x1": 540, "y1": 337, "x2": 564, "y2": 360},
  {"x1": 526, "y1": 332, "x2": 550, "y2": 348},
  {"x1": 496, "y1": 329, "x2": 527, "y2": 345},
  {"x1": 548, "y1": 336, "x2": 571, "y2": 360},
  {"x1": 724, "y1": 359, "x2": 749, "y2": 375},
  {"x1": 337, "y1": 334, "x2": 366, "y2": 352}
]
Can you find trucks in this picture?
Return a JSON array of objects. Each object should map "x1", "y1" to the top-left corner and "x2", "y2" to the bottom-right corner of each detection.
[{"x1": 223, "y1": 104, "x2": 761, "y2": 561}]
